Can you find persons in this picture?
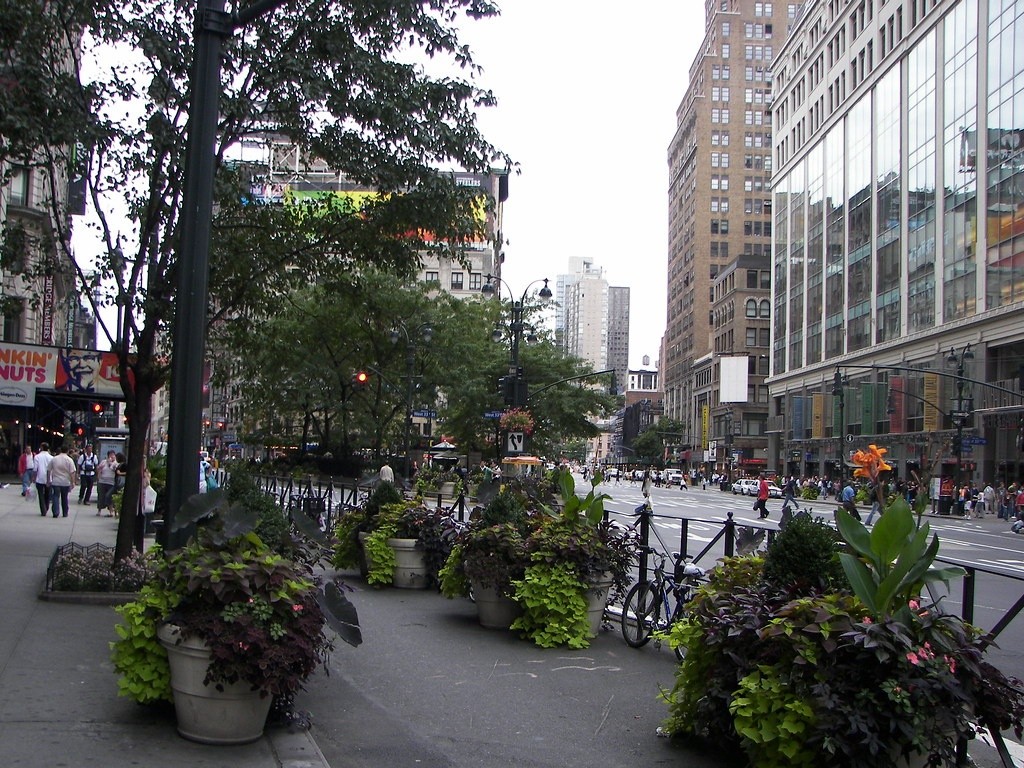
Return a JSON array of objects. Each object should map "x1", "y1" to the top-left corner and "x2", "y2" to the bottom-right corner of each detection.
[
  {"x1": 410, "y1": 460, "x2": 467, "y2": 476},
  {"x1": 583, "y1": 464, "x2": 637, "y2": 486},
  {"x1": 134, "y1": 456, "x2": 152, "y2": 557},
  {"x1": 47, "y1": 446, "x2": 77, "y2": 518},
  {"x1": 780, "y1": 476, "x2": 800, "y2": 510},
  {"x1": 654, "y1": 469, "x2": 724, "y2": 490},
  {"x1": 150, "y1": 426, "x2": 169, "y2": 454},
  {"x1": 478, "y1": 460, "x2": 502, "y2": 487},
  {"x1": 19, "y1": 443, "x2": 85, "y2": 496},
  {"x1": 378, "y1": 460, "x2": 394, "y2": 482},
  {"x1": 34, "y1": 443, "x2": 52, "y2": 516},
  {"x1": 756, "y1": 474, "x2": 770, "y2": 519},
  {"x1": 524, "y1": 460, "x2": 568, "y2": 478},
  {"x1": 224, "y1": 454, "x2": 261, "y2": 464},
  {"x1": 865, "y1": 481, "x2": 884, "y2": 526},
  {"x1": 77, "y1": 444, "x2": 98, "y2": 505},
  {"x1": 97, "y1": 449, "x2": 127, "y2": 514},
  {"x1": 641, "y1": 471, "x2": 654, "y2": 518},
  {"x1": 780, "y1": 474, "x2": 922, "y2": 501},
  {"x1": 953, "y1": 481, "x2": 1024, "y2": 534},
  {"x1": 842, "y1": 478, "x2": 865, "y2": 525},
  {"x1": 200, "y1": 454, "x2": 218, "y2": 493}
]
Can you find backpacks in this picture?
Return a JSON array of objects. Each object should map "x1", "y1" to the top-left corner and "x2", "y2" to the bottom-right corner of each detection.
[{"x1": 202, "y1": 461, "x2": 217, "y2": 492}]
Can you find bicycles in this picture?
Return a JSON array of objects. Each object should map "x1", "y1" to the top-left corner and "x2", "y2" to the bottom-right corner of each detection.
[{"x1": 621, "y1": 546, "x2": 706, "y2": 663}]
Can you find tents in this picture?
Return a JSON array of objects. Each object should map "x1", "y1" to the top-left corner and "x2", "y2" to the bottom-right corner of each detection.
[{"x1": 424, "y1": 440, "x2": 467, "y2": 471}]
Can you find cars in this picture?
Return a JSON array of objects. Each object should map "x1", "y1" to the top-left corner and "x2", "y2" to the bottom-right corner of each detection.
[
  {"x1": 731, "y1": 479, "x2": 754, "y2": 495},
  {"x1": 748, "y1": 479, "x2": 782, "y2": 498},
  {"x1": 622, "y1": 470, "x2": 663, "y2": 482},
  {"x1": 607, "y1": 468, "x2": 622, "y2": 477},
  {"x1": 546, "y1": 464, "x2": 590, "y2": 473}
]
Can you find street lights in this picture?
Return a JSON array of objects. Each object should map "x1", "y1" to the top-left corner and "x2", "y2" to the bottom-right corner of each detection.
[
  {"x1": 481, "y1": 274, "x2": 553, "y2": 410},
  {"x1": 390, "y1": 320, "x2": 434, "y2": 489},
  {"x1": 947, "y1": 342, "x2": 974, "y2": 515},
  {"x1": 618, "y1": 451, "x2": 621, "y2": 469}
]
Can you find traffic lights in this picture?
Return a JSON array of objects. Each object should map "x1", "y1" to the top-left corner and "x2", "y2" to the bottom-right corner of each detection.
[
  {"x1": 498, "y1": 374, "x2": 507, "y2": 404},
  {"x1": 950, "y1": 434, "x2": 959, "y2": 455},
  {"x1": 353, "y1": 370, "x2": 368, "y2": 393},
  {"x1": 205, "y1": 420, "x2": 209, "y2": 428},
  {"x1": 220, "y1": 423, "x2": 222, "y2": 430}
]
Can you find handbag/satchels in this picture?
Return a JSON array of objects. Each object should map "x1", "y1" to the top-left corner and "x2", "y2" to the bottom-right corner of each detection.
[
  {"x1": 25, "y1": 483, "x2": 38, "y2": 503},
  {"x1": 753, "y1": 501, "x2": 758, "y2": 511},
  {"x1": 142, "y1": 485, "x2": 157, "y2": 514}
]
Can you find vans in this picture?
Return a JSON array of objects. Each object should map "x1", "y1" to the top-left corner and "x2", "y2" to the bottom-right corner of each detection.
[
  {"x1": 201, "y1": 451, "x2": 211, "y2": 464},
  {"x1": 151, "y1": 443, "x2": 168, "y2": 457}
]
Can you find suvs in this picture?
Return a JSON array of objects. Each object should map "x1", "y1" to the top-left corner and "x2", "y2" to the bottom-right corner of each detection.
[{"x1": 663, "y1": 469, "x2": 682, "y2": 485}]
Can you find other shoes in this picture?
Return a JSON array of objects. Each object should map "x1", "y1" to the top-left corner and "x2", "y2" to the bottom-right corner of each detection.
[
  {"x1": 967, "y1": 516, "x2": 971, "y2": 519},
  {"x1": 78, "y1": 498, "x2": 82, "y2": 504},
  {"x1": 962, "y1": 515, "x2": 967, "y2": 518},
  {"x1": 84, "y1": 501, "x2": 90, "y2": 505}
]
